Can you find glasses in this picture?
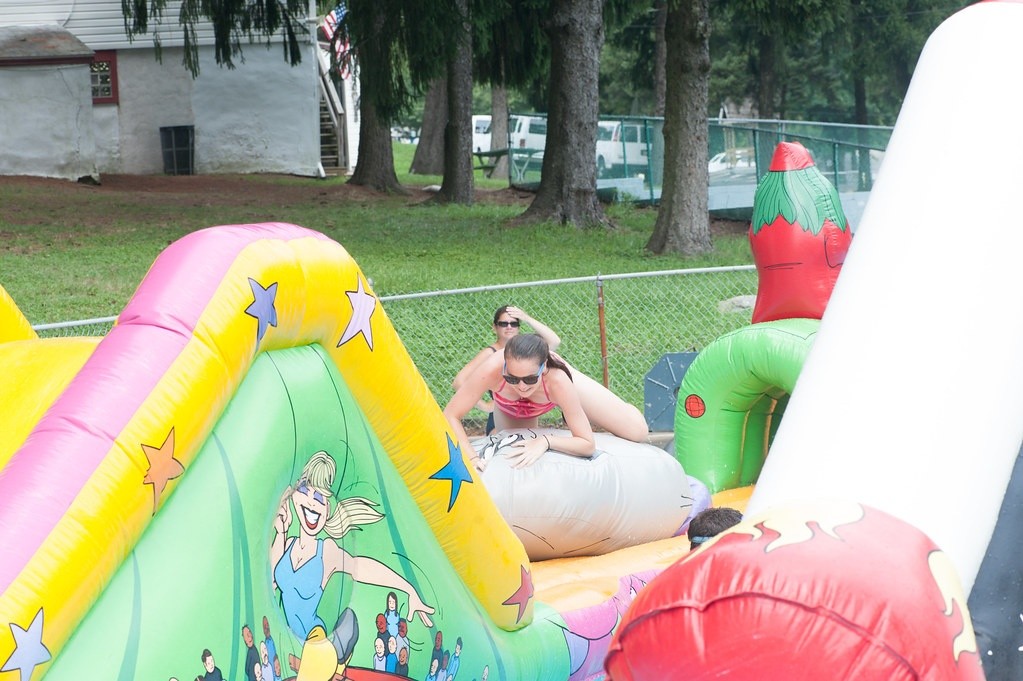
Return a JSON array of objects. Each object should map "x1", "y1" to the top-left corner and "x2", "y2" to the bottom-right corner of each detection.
[
  {"x1": 496, "y1": 322, "x2": 520, "y2": 327},
  {"x1": 502, "y1": 360, "x2": 547, "y2": 385}
]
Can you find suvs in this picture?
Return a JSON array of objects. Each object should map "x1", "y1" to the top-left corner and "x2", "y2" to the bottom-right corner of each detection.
[
  {"x1": 595, "y1": 120, "x2": 654, "y2": 179},
  {"x1": 508, "y1": 114, "x2": 548, "y2": 164}
]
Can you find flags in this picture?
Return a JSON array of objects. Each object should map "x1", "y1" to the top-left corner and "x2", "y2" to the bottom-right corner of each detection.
[{"x1": 322, "y1": 0, "x2": 351, "y2": 80}]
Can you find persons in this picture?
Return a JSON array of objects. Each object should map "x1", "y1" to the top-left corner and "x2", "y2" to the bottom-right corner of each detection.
[
  {"x1": 443, "y1": 333, "x2": 648, "y2": 471},
  {"x1": 451, "y1": 304, "x2": 561, "y2": 436},
  {"x1": 688, "y1": 508, "x2": 742, "y2": 551}
]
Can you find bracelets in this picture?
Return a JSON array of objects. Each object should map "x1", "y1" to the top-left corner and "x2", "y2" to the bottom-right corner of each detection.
[
  {"x1": 543, "y1": 435, "x2": 551, "y2": 450},
  {"x1": 470, "y1": 456, "x2": 478, "y2": 461}
]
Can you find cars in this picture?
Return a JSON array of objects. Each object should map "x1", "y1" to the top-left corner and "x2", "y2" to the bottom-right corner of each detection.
[{"x1": 707, "y1": 148, "x2": 757, "y2": 173}]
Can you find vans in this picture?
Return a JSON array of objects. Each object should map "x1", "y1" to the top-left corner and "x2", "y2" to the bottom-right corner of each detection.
[{"x1": 471, "y1": 115, "x2": 492, "y2": 154}]
